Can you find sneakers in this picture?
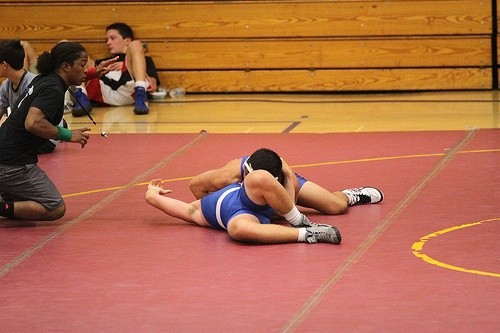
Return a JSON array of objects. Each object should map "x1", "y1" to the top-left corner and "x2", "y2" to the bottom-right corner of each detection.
[
  {"x1": 307, "y1": 222, "x2": 341, "y2": 244},
  {"x1": 341, "y1": 187, "x2": 384, "y2": 206},
  {"x1": 290, "y1": 214, "x2": 310, "y2": 228},
  {"x1": 134, "y1": 86, "x2": 149, "y2": 115},
  {"x1": 72, "y1": 88, "x2": 92, "y2": 116}
]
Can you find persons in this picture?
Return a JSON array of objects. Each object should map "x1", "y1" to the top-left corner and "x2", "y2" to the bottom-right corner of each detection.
[
  {"x1": 0, "y1": 40, "x2": 38, "y2": 126},
  {"x1": 0, "y1": 42, "x2": 92, "y2": 222},
  {"x1": 189, "y1": 148, "x2": 384, "y2": 214},
  {"x1": 72, "y1": 23, "x2": 159, "y2": 118},
  {"x1": 146, "y1": 170, "x2": 342, "y2": 244},
  {"x1": 20, "y1": 39, "x2": 94, "y2": 116}
]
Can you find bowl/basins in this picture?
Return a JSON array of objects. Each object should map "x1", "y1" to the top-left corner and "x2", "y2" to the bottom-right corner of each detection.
[{"x1": 151, "y1": 88, "x2": 166, "y2": 99}]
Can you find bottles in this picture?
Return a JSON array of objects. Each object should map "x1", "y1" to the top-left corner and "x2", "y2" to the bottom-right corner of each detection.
[{"x1": 169, "y1": 87, "x2": 186, "y2": 98}]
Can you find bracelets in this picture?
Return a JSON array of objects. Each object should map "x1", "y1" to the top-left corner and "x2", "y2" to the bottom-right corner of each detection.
[{"x1": 55, "y1": 125, "x2": 73, "y2": 141}]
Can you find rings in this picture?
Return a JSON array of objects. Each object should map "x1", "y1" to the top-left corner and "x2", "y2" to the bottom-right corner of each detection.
[{"x1": 81, "y1": 141, "x2": 84, "y2": 143}]
